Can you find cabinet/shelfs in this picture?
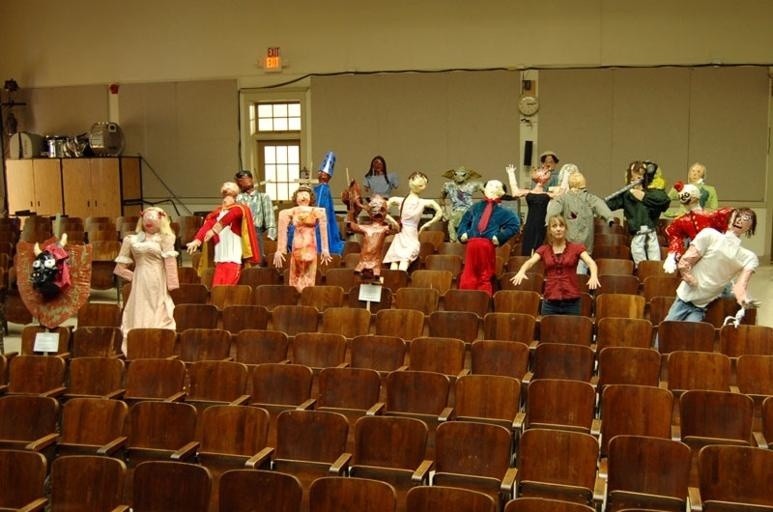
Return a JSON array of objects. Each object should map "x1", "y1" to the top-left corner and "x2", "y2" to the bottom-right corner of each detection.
[
  {"x1": 4, "y1": 156, "x2": 65, "y2": 231},
  {"x1": 62, "y1": 156, "x2": 144, "y2": 232}
]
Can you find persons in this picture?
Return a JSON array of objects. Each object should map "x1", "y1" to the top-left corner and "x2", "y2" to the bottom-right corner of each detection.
[
  {"x1": 668, "y1": 164, "x2": 719, "y2": 213},
  {"x1": 664, "y1": 208, "x2": 763, "y2": 321},
  {"x1": 187, "y1": 152, "x2": 561, "y2": 292},
  {"x1": 113, "y1": 205, "x2": 178, "y2": 356},
  {"x1": 605, "y1": 162, "x2": 676, "y2": 265},
  {"x1": 546, "y1": 164, "x2": 614, "y2": 274},
  {"x1": 663, "y1": 183, "x2": 733, "y2": 274},
  {"x1": 511, "y1": 215, "x2": 602, "y2": 316}
]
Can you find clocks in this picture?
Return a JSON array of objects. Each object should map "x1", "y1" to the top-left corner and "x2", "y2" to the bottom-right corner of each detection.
[{"x1": 517, "y1": 94, "x2": 540, "y2": 116}]
